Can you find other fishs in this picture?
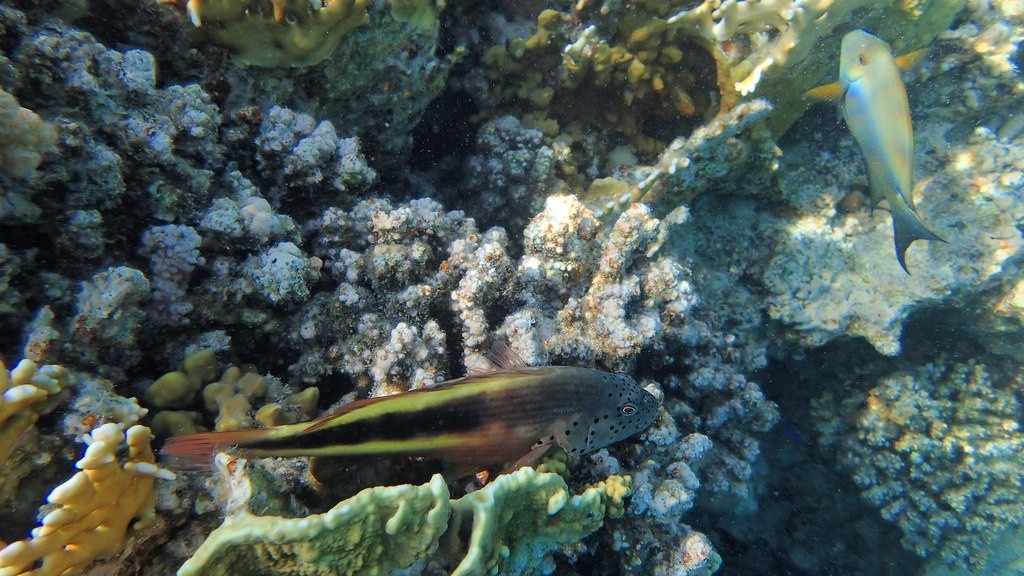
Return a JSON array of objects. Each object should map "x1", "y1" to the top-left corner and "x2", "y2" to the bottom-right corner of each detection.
[
  {"x1": 807, "y1": 28, "x2": 949, "y2": 277},
  {"x1": 162, "y1": 362, "x2": 658, "y2": 465}
]
